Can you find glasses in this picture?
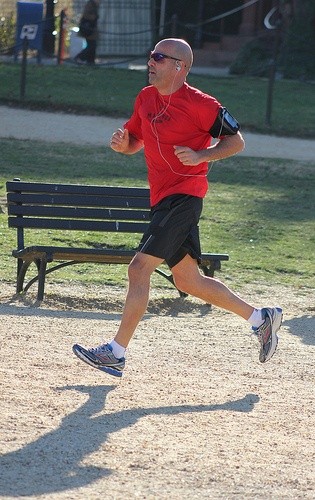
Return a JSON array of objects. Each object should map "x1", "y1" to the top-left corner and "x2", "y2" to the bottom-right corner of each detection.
[{"x1": 151, "y1": 53, "x2": 182, "y2": 62}]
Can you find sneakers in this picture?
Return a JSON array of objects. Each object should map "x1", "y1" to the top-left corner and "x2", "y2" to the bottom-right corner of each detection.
[
  {"x1": 251, "y1": 307, "x2": 283, "y2": 363},
  {"x1": 72, "y1": 343, "x2": 125, "y2": 377}
]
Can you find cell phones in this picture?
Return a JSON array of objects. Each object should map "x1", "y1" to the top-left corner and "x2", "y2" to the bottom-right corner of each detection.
[{"x1": 220, "y1": 108, "x2": 240, "y2": 131}]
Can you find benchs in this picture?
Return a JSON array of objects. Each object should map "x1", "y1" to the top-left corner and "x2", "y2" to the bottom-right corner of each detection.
[{"x1": 5, "y1": 177, "x2": 230, "y2": 309}]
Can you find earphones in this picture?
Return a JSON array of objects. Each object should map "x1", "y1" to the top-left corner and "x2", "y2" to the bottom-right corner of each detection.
[{"x1": 176, "y1": 66, "x2": 181, "y2": 71}]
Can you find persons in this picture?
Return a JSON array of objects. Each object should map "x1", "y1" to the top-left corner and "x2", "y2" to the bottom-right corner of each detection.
[{"x1": 72, "y1": 39, "x2": 283, "y2": 377}]
